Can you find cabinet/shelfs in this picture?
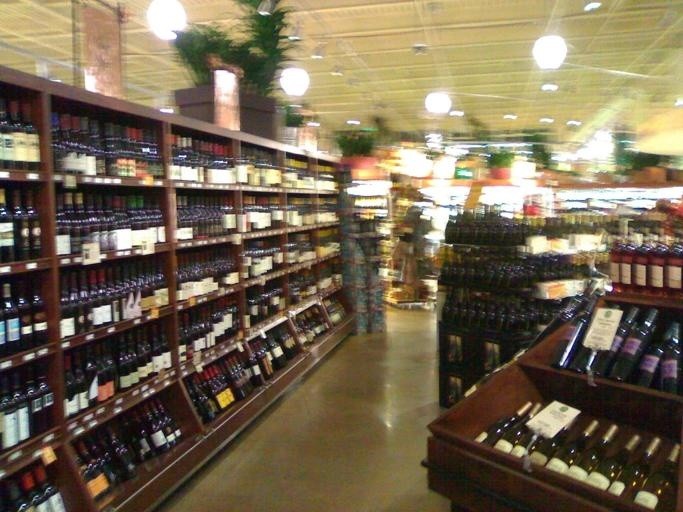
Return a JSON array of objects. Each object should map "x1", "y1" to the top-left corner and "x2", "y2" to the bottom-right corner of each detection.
[
  {"x1": 421, "y1": 283, "x2": 681, "y2": 512},
  {"x1": 356, "y1": 182, "x2": 427, "y2": 309},
  {"x1": 0, "y1": 67, "x2": 357, "y2": 512},
  {"x1": 439, "y1": 197, "x2": 683, "y2": 411}
]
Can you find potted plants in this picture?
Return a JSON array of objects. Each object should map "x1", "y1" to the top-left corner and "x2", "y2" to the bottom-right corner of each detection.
[
  {"x1": 337, "y1": 127, "x2": 378, "y2": 179},
  {"x1": 486, "y1": 149, "x2": 514, "y2": 180},
  {"x1": 173, "y1": 0, "x2": 297, "y2": 141}
]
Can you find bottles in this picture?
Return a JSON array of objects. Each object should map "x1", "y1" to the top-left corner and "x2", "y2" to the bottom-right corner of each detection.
[
  {"x1": 632, "y1": 245, "x2": 648, "y2": 290},
  {"x1": 587, "y1": 435, "x2": 642, "y2": 490},
  {"x1": 512, "y1": 427, "x2": 538, "y2": 458},
  {"x1": 77, "y1": 437, "x2": 111, "y2": 496},
  {"x1": 632, "y1": 443, "x2": 681, "y2": 510},
  {"x1": 620, "y1": 244, "x2": 631, "y2": 290},
  {"x1": 24, "y1": 361, "x2": 44, "y2": 436},
  {"x1": 95, "y1": 340, "x2": 107, "y2": 405},
  {"x1": 322, "y1": 258, "x2": 349, "y2": 294},
  {"x1": 64, "y1": 191, "x2": 80, "y2": 255},
  {"x1": 180, "y1": 296, "x2": 250, "y2": 359},
  {"x1": 59, "y1": 270, "x2": 73, "y2": 340},
  {"x1": 6, "y1": 480, "x2": 31, "y2": 512},
  {"x1": 11, "y1": 368, "x2": 30, "y2": 443},
  {"x1": 128, "y1": 408, "x2": 155, "y2": 458},
  {"x1": 635, "y1": 333, "x2": 660, "y2": 387},
  {"x1": 65, "y1": 352, "x2": 80, "y2": 417},
  {"x1": 74, "y1": 347, "x2": 91, "y2": 410},
  {"x1": 88, "y1": 432, "x2": 118, "y2": 486},
  {"x1": 245, "y1": 243, "x2": 286, "y2": 278},
  {"x1": 33, "y1": 276, "x2": 49, "y2": 346},
  {"x1": 89, "y1": 269, "x2": 104, "y2": 329},
  {"x1": 172, "y1": 133, "x2": 236, "y2": 185},
  {"x1": 531, "y1": 410, "x2": 580, "y2": 466},
  {"x1": 12, "y1": 186, "x2": 29, "y2": 261},
  {"x1": 126, "y1": 329, "x2": 141, "y2": 386},
  {"x1": 71, "y1": 115, "x2": 83, "y2": 175},
  {"x1": 559, "y1": 301, "x2": 598, "y2": 367},
  {"x1": 58, "y1": 113, "x2": 73, "y2": 176},
  {"x1": 609, "y1": 308, "x2": 660, "y2": 383},
  {"x1": 184, "y1": 355, "x2": 262, "y2": 418},
  {"x1": 248, "y1": 277, "x2": 286, "y2": 324},
  {"x1": 661, "y1": 321, "x2": 682, "y2": 394},
  {"x1": 649, "y1": 243, "x2": 666, "y2": 292},
  {"x1": 241, "y1": 322, "x2": 300, "y2": 384},
  {"x1": 437, "y1": 241, "x2": 605, "y2": 292},
  {"x1": 494, "y1": 403, "x2": 545, "y2": 453},
  {"x1": 121, "y1": 414, "x2": 147, "y2": 462},
  {"x1": 54, "y1": 192, "x2": 70, "y2": 256},
  {"x1": 0, "y1": 186, "x2": 15, "y2": 263},
  {"x1": 316, "y1": 165, "x2": 344, "y2": 188},
  {"x1": 18, "y1": 280, "x2": 33, "y2": 351},
  {"x1": 317, "y1": 229, "x2": 343, "y2": 258},
  {"x1": 139, "y1": 401, "x2": 169, "y2": 456},
  {"x1": 444, "y1": 199, "x2": 604, "y2": 244},
  {"x1": 244, "y1": 195, "x2": 285, "y2": 232},
  {"x1": 0, "y1": 95, "x2": 15, "y2": 171},
  {"x1": 667, "y1": 244, "x2": 683, "y2": 292},
  {"x1": 153, "y1": 393, "x2": 183, "y2": 444},
  {"x1": 108, "y1": 269, "x2": 171, "y2": 321},
  {"x1": 295, "y1": 305, "x2": 328, "y2": 346},
  {"x1": 80, "y1": 115, "x2": 97, "y2": 176},
  {"x1": 107, "y1": 421, "x2": 136, "y2": 481},
  {"x1": 145, "y1": 320, "x2": 164, "y2": 374},
  {"x1": 105, "y1": 191, "x2": 117, "y2": 251},
  {"x1": 97, "y1": 192, "x2": 108, "y2": 252},
  {"x1": 292, "y1": 268, "x2": 322, "y2": 303},
  {"x1": 0, "y1": 296, "x2": 7, "y2": 359},
  {"x1": 107, "y1": 121, "x2": 164, "y2": 180},
  {"x1": 69, "y1": 270, "x2": 84, "y2": 336},
  {"x1": 99, "y1": 267, "x2": 112, "y2": 328},
  {"x1": 569, "y1": 304, "x2": 622, "y2": 372},
  {"x1": 322, "y1": 199, "x2": 341, "y2": 225},
  {"x1": 283, "y1": 155, "x2": 316, "y2": 187},
  {"x1": 605, "y1": 211, "x2": 674, "y2": 240},
  {"x1": 545, "y1": 419, "x2": 600, "y2": 474},
  {"x1": 3, "y1": 283, "x2": 20, "y2": 352},
  {"x1": 102, "y1": 339, "x2": 116, "y2": 401},
  {"x1": 324, "y1": 295, "x2": 349, "y2": 324},
  {"x1": 239, "y1": 147, "x2": 284, "y2": 186},
  {"x1": 117, "y1": 332, "x2": 131, "y2": 393},
  {"x1": 289, "y1": 234, "x2": 316, "y2": 266},
  {"x1": 21, "y1": 469, "x2": 50, "y2": 512},
  {"x1": 87, "y1": 190, "x2": 99, "y2": 243},
  {"x1": 23, "y1": 95, "x2": 41, "y2": 171},
  {"x1": 24, "y1": 183, "x2": 41, "y2": 258},
  {"x1": 289, "y1": 197, "x2": 321, "y2": 226},
  {"x1": 609, "y1": 241, "x2": 620, "y2": 288},
  {"x1": 173, "y1": 193, "x2": 239, "y2": 240},
  {"x1": 158, "y1": 319, "x2": 172, "y2": 369},
  {"x1": 80, "y1": 271, "x2": 94, "y2": 334},
  {"x1": 75, "y1": 192, "x2": 90, "y2": 246},
  {"x1": 143, "y1": 324, "x2": 154, "y2": 377},
  {"x1": 607, "y1": 437, "x2": 663, "y2": 496},
  {"x1": 68, "y1": 445, "x2": 102, "y2": 502},
  {"x1": 116, "y1": 194, "x2": 168, "y2": 248},
  {"x1": 568, "y1": 424, "x2": 619, "y2": 481},
  {"x1": 9, "y1": 95, "x2": 27, "y2": 171},
  {"x1": 147, "y1": 397, "x2": 177, "y2": 448},
  {"x1": 36, "y1": 360, "x2": 55, "y2": 432},
  {"x1": 86, "y1": 344, "x2": 98, "y2": 408},
  {"x1": 438, "y1": 288, "x2": 565, "y2": 336},
  {"x1": 0, "y1": 372, "x2": 19, "y2": 453},
  {"x1": 94, "y1": 115, "x2": 107, "y2": 175},
  {"x1": 135, "y1": 326, "x2": 148, "y2": 380},
  {"x1": 51, "y1": 114, "x2": 62, "y2": 175},
  {"x1": 173, "y1": 255, "x2": 240, "y2": 300},
  {"x1": 582, "y1": 306, "x2": 641, "y2": 375},
  {"x1": 96, "y1": 427, "x2": 125, "y2": 485},
  {"x1": 473, "y1": 400, "x2": 532, "y2": 445},
  {"x1": 33, "y1": 461, "x2": 66, "y2": 512}
]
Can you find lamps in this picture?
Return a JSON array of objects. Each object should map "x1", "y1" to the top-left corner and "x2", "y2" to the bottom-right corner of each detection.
[
  {"x1": 584, "y1": 1, "x2": 602, "y2": 11},
  {"x1": 542, "y1": 80, "x2": 559, "y2": 91},
  {"x1": 531, "y1": 34, "x2": 569, "y2": 71},
  {"x1": 279, "y1": 66, "x2": 310, "y2": 98},
  {"x1": 313, "y1": 46, "x2": 325, "y2": 60},
  {"x1": 425, "y1": 92, "x2": 452, "y2": 114},
  {"x1": 145, "y1": 1, "x2": 188, "y2": 41},
  {"x1": 257, "y1": 1, "x2": 273, "y2": 15},
  {"x1": 288, "y1": 25, "x2": 302, "y2": 41}
]
